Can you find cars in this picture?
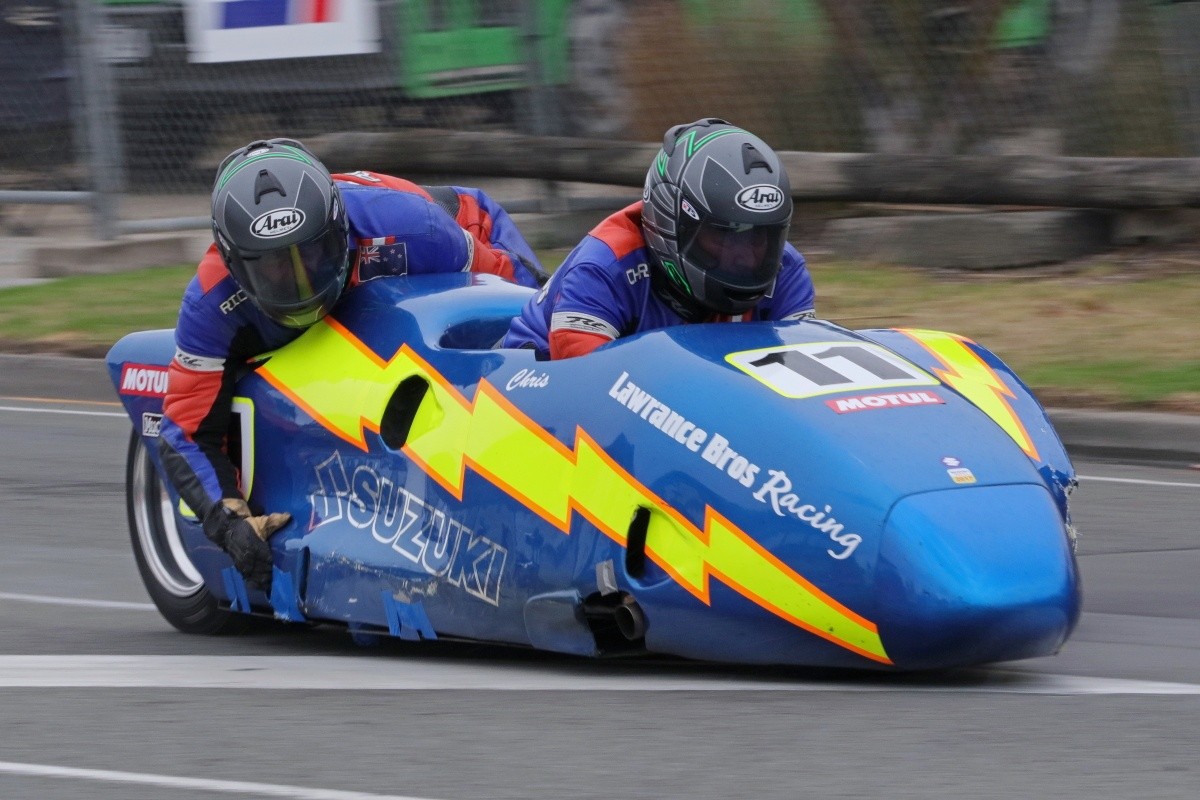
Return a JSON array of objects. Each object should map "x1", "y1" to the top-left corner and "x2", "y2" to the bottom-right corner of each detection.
[{"x1": 1, "y1": 0, "x2": 1200, "y2": 195}]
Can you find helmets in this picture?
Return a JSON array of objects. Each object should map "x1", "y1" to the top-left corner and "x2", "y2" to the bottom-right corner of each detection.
[
  {"x1": 211, "y1": 137, "x2": 350, "y2": 329},
  {"x1": 641, "y1": 118, "x2": 793, "y2": 316}
]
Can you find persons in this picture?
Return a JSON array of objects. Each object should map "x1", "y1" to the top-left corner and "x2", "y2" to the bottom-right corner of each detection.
[
  {"x1": 499, "y1": 117, "x2": 816, "y2": 361},
  {"x1": 158, "y1": 138, "x2": 552, "y2": 592}
]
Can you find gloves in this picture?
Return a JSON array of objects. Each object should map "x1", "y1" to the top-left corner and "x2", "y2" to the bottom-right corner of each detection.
[{"x1": 202, "y1": 497, "x2": 291, "y2": 592}]
